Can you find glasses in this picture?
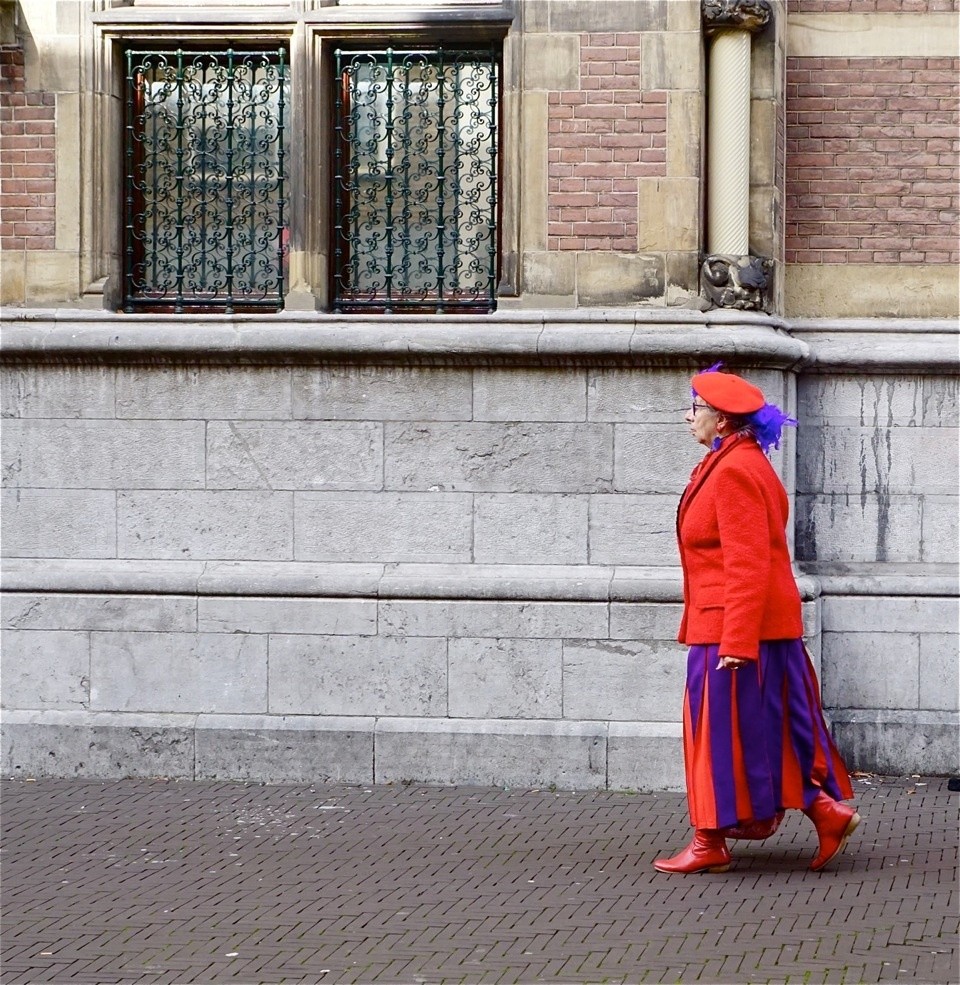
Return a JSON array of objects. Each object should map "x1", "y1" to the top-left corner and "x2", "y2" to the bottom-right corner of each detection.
[{"x1": 692, "y1": 402, "x2": 709, "y2": 416}]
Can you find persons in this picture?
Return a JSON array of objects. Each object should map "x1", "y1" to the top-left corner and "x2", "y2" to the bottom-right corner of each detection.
[{"x1": 653, "y1": 363, "x2": 860, "y2": 874}]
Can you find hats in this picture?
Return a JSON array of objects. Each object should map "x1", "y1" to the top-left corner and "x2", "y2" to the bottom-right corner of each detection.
[{"x1": 692, "y1": 362, "x2": 799, "y2": 455}]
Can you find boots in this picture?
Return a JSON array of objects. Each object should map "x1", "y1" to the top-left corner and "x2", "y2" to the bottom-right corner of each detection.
[
  {"x1": 654, "y1": 826, "x2": 732, "y2": 874},
  {"x1": 799, "y1": 789, "x2": 862, "y2": 872}
]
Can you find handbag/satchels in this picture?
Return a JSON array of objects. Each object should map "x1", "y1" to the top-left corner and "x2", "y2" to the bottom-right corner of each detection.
[{"x1": 725, "y1": 807, "x2": 786, "y2": 840}]
